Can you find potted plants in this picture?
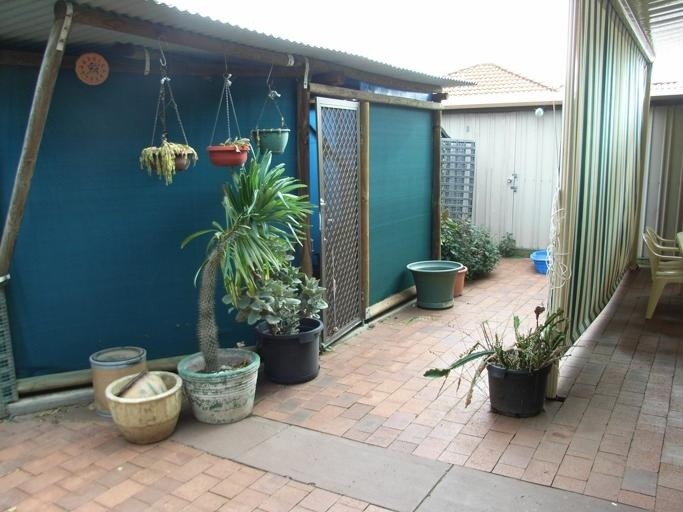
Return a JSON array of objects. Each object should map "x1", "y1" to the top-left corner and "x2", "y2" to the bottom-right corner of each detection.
[
  {"x1": 422, "y1": 305, "x2": 586, "y2": 419},
  {"x1": 220, "y1": 250, "x2": 330, "y2": 384},
  {"x1": 175, "y1": 150, "x2": 313, "y2": 423},
  {"x1": 137, "y1": 128, "x2": 256, "y2": 187}
]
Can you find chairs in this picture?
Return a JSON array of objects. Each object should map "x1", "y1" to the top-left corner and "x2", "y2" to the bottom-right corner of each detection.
[{"x1": 641, "y1": 227, "x2": 683, "y2": 320}]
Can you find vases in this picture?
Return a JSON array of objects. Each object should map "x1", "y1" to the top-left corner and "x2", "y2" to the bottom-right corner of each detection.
[
  {"x1": 249, "y1": 128, "x2": 291, "y2": 154},
  {"x1": 406, "y1": 260, "x2": 468, "y2": 309},
  {"x1": 89, "y1": 346, "x2": 183, "y2": 444}
]
